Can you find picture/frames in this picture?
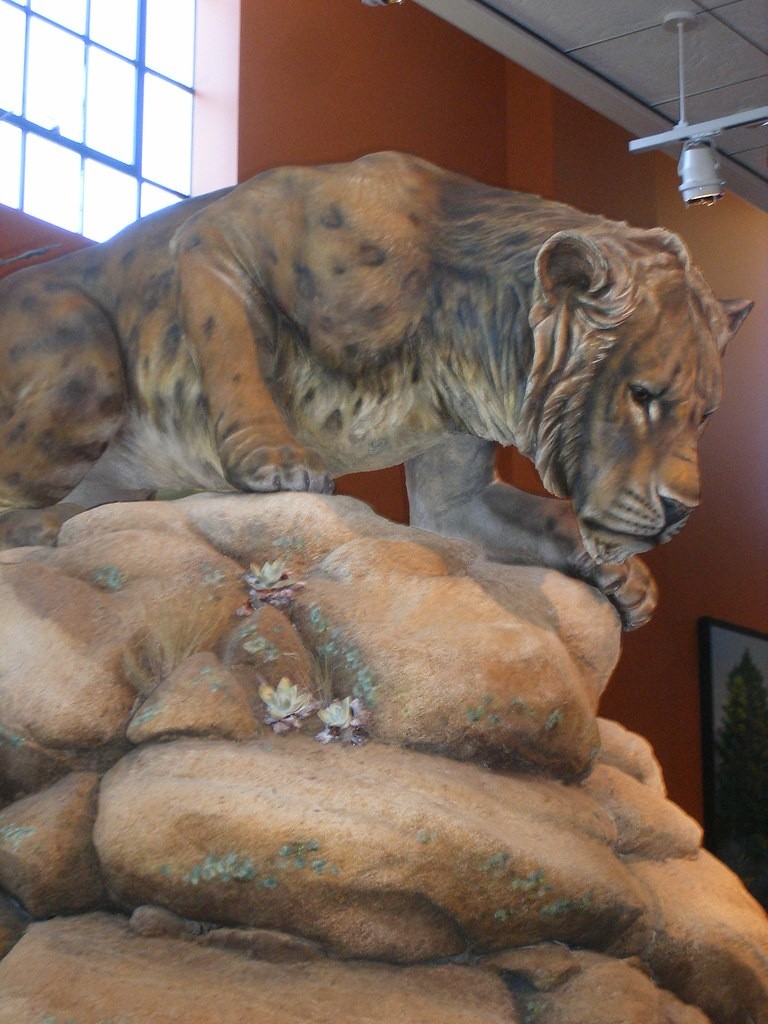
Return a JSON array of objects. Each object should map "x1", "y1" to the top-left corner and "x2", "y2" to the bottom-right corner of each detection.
[{"x1": 696, "y1": 618, "x2": 768, "y2": 892}]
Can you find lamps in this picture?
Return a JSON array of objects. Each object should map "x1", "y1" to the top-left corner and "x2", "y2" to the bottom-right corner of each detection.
[
  {"x1": 626, "y1": 11, "x2": 768, "y2": 151},
  {"x1": 675, "y1": 132, "x2": 726, "y2": 209}
]
